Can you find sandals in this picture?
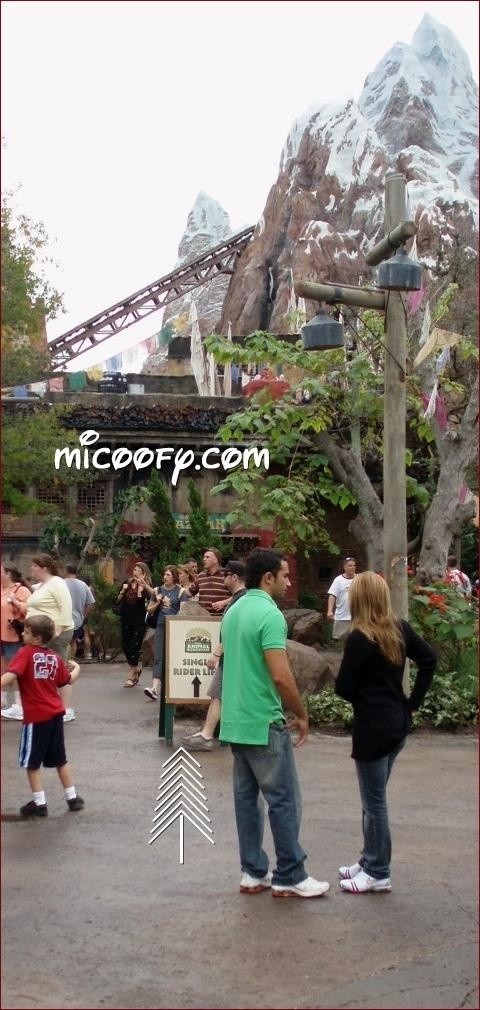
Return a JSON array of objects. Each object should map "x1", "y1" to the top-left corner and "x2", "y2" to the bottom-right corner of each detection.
[{"x1": 124, "y1": 675, "x2": 139, "y2": 688}]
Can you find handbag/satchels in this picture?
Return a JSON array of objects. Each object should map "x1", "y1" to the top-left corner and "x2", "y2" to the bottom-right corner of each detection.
[
  {"x1": 143, "y1": 587, "x2": 162, "y2": 629},
  {"x1": 11, "y1": 618, "x2": 28, "y2": 640},
  {"x1": 110, "y1": 578, "x2": 132, "y2": 616}
]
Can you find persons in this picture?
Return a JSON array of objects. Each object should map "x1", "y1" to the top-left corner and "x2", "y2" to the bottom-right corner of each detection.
[
  {"x1": 334, "y1": 571, "x2": 437, "y2": 894},
  {"x1": 0, "y1": 614, "x2": 85, "y2": 815},
  {"x1": 0, "y1": 552, "x2": 95, "y2": 722},
  {"x1": 114, "y1": 544, "x2": 247, "y2": 750},
  {"x1": 407, "y1": 553, "x2": 417, "y2": 579},
  {"x1": 327, "y1": 557, "x2": 359, "y2": 640},
  {"x1": 219, "y1": 547, "x2": 332, "y2": 897},
  {"x1": 446, "y1": 556, "x2": 472, "y2": 602}
]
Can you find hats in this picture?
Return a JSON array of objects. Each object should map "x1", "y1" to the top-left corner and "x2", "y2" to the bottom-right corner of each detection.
[{"x1": 219, "y1": 560, "x2": 247, "y2": 577}]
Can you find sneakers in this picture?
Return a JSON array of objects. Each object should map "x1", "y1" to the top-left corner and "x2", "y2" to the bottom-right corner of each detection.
[
  {"x1": 339, "y1": 863, "x2": 359, "y2": 878},
  {"x1": 1, "y1": 704, "x2": 24, "y2": 721},
  {"x1": 239, "y1": 870, "x2": 276, "y2": 895},
  {"x1": 65, "y1": 793, "x2": 85, "y2": 810},
  {"x1": 339, "y1": 871, "x2": 392, "y2": 893},
  {"x1": 269, "y1": 874, "x2": 330, "y2": 899},
  {"x1": 143, "y1": 687, "x2": 158, "y2": 700},
  {"x1": 181, "y1": 731, "x2": 214, "y2": 751},
  {"x1": 20, "y1": 799, "x2": 49, "y2": 817},
  {"x1": 62, "y1": 708, "x2": 76, "y2": 722}
]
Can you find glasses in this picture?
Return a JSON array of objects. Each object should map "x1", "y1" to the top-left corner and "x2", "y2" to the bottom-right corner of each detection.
[{"x1": 224, "y1": 572, "x2": 233, "y2": 577}]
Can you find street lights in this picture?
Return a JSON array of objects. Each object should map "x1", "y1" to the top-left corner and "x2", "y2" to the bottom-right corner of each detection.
[{"x1": 292, "y1": 170, "x2": 424, "y2": 628}]
